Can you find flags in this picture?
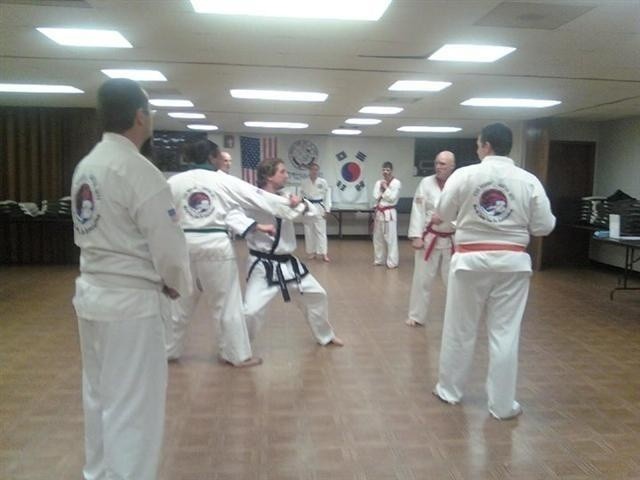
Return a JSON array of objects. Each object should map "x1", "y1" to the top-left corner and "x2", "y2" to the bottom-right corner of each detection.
[
  {"x1": 239, "y1": 135, "x2": 280, "y2": 189},
  {"x1": 329, "y1": 141, "x2": 370, "y2": 203}
]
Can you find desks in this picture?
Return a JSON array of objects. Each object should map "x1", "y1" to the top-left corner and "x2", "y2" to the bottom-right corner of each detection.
[
  {"x1": 590, "y1": 234, "x2": 639, "y2": 303},
  {"x1": 323, "y1": 204, "x2": 379, "y2": 244}
]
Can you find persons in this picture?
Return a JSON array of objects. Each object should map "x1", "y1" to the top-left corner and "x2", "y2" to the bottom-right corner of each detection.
[
  {"x1": 216, "y1": 157, "x2": 344, "y2": 363},
  {"x1": 404, "y1": 149, "x2": 456, "y2": 326},
  {"x1": 217, "y1": 150, "x2": 232, "y2": 174},
  {"x1": 370, "y1": 161, "x2": 401, "y2": 270},
  {"x1": 300, "y1": 162, "x2": 332, "y2": 262},
  {"x1": 416, "y1": 123, "x2": 556, "y2": 419},
  {"x1": 167, "y1": 138, "x2": 302, "y2": 369},
  {"x1": 72, "y1": 76, "x2": 195, "y2": 479}
]
